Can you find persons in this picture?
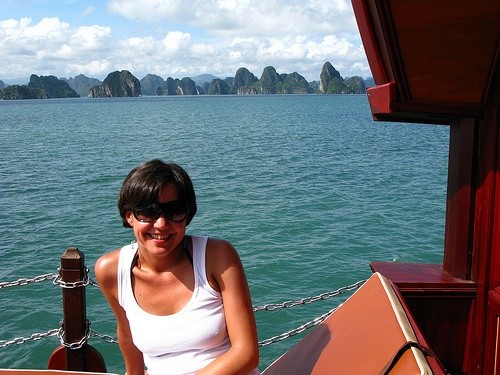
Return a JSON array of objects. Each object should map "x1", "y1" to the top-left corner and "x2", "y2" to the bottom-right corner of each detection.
[{"x1": 94, "y1": 157, "x2": 262, "y2": 375}]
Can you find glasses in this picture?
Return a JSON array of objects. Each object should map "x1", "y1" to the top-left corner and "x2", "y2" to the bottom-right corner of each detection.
[{"x1": 127, "y1": 201, "x2": 193, "y2": 223}]
